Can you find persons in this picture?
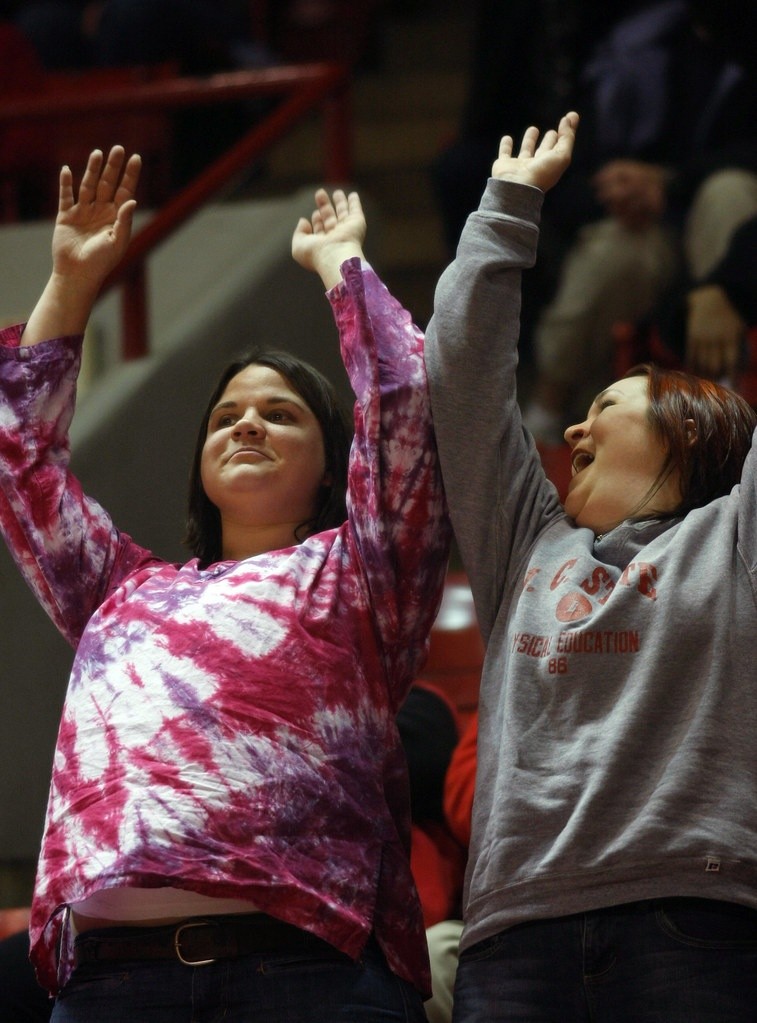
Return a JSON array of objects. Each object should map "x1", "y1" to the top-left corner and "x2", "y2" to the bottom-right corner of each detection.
[
  {"x1": 517, "y1": 24, "x2": 756, "y2": 447},
  {"x1": 425, "y1": 110, "x2": 757, "y2": 1023},
  {"x1": 659, "y1": 140, "x2": 754, "y2": 385},
  {"x1": 0, "y1": 144, "x2": 457, "y2": 1023}
]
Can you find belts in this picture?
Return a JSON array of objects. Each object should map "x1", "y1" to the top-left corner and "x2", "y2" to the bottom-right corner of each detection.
[{"x1": 68, "y1": 915, "x2": 384, "y2": 975}]
